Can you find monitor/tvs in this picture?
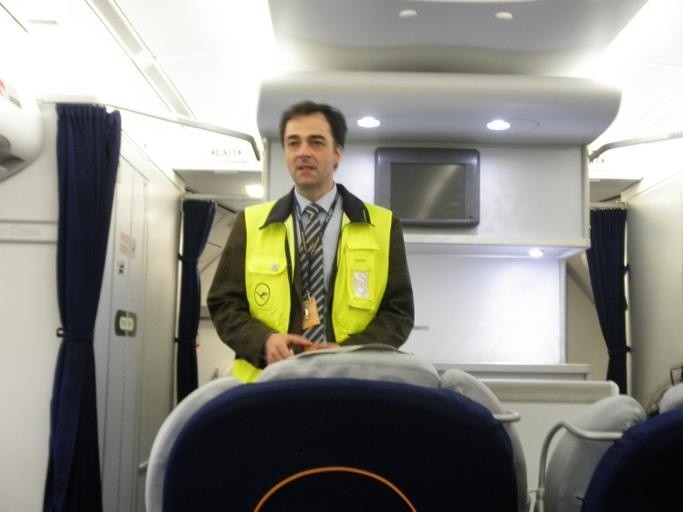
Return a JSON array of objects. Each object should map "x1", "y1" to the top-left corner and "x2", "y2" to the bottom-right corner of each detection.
[{"x1": 374, "y1": 146, "x2": 480, "y2": 228}]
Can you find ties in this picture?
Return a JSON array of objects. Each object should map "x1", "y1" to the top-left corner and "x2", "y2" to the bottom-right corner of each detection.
[{"x1": 299, "y1": 204, "x2": 326, "y2": 344}]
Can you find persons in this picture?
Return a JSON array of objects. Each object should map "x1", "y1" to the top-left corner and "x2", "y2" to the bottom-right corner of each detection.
[{"x1": 205, "y1": 98, "x2": 415, "y2": 384}]
